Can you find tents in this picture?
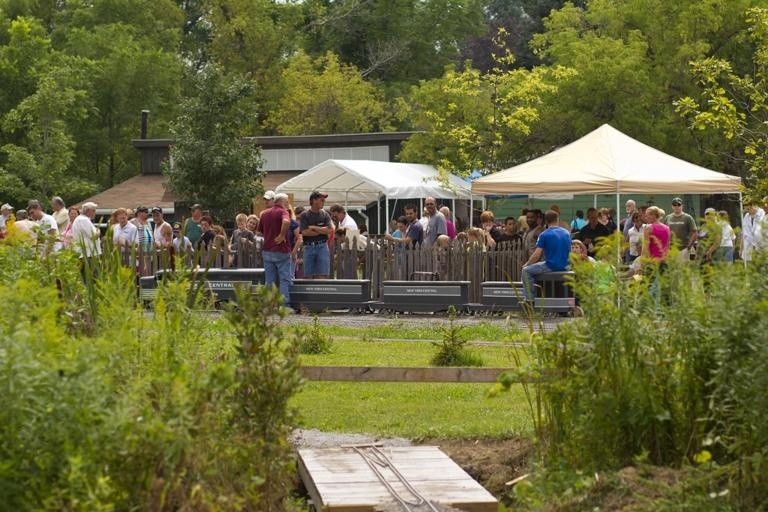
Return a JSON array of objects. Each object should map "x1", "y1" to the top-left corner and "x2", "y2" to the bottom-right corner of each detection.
[{"x1": 470, "y1": 123, "x2": 744, "y2": 309}]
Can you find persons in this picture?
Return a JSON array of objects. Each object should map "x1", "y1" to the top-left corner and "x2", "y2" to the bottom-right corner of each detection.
[
  {"x1": 1, "y1": 195, "x2": 264, "y2": 287},
  {"x1": 258, "y1": 189, "x2": 379, "y2": 308},
  {"x1": 378, "y1": 196, "x2": 457, "y2": 281},
  {"x1": 457, "y1": 196, "x2": 768, "y2": 309}
]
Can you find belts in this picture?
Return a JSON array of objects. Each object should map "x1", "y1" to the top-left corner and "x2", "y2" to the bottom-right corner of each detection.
[{"x1": 304, "y1": 240, "x2": 327, "y2": 246}]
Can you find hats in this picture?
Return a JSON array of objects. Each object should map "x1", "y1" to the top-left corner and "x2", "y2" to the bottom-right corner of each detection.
[
  {"x1": 704, "y1": 207, "x2": 716, "y2": 215},
  {"x1": 310, "y1": 190, "x2": 329, "y2": 199},
  {"x1": 134, "y1": 206, "x2": 182, "y2": 232},
  {"x1": 263, "y1": 190, "x2": 276, "y2": 200},
  {"x1": 1, "y1": 203, "x2": 26, "y2": 219},
  {"x1": 671, "y1": 197, "x2": 683, "y2": 205}
]
